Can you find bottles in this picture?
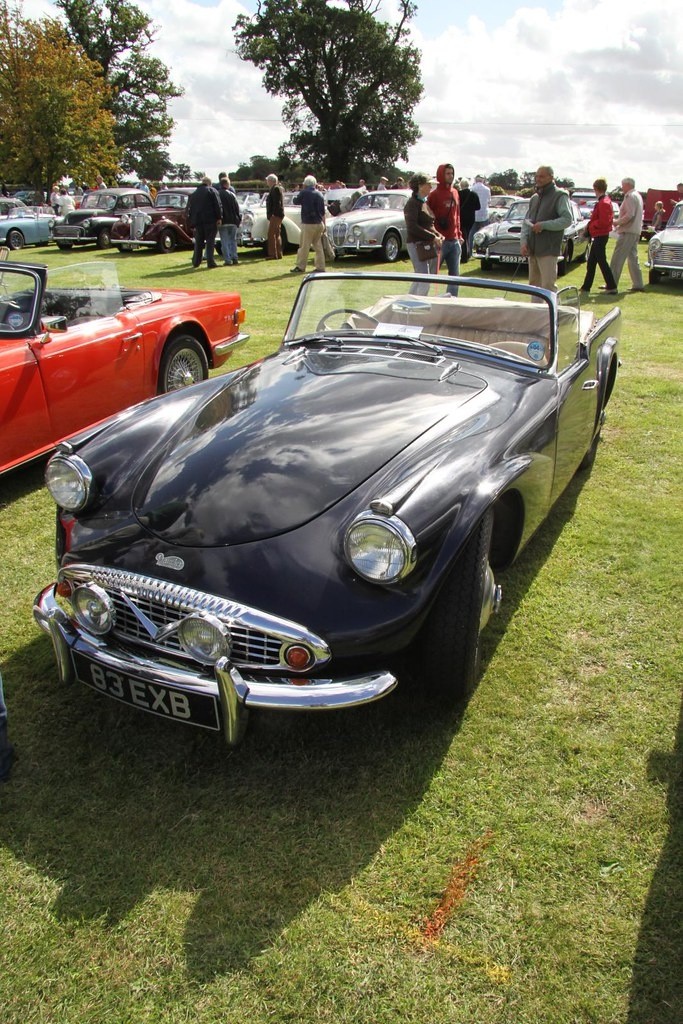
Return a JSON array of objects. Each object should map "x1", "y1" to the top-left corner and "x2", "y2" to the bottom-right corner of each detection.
[{"x1": 647, "y1": 226, "x2": 657, "y2": 235}]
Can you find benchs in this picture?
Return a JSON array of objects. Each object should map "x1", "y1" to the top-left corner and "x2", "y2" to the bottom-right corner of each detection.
[
  {"x1": 0, "y1": 287, "x2": 155, "y2": 327},
  {"x1": 351, "y1": 294, "x2": 583, "y2": 367}
]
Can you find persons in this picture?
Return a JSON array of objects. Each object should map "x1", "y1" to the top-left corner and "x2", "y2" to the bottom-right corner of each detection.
[
  {"x1": 335, "y1": 181, "x2": 347, "y2": 189},
  {"x1": 454, "y1": 174, "x2": 491, "y2": 264},
  {"x1": 670, "y1": 182, "x2": 683, "y2": 220},
  {"x1": 187, "y1": 176, "x2": 222, "y2": 268},
  {"x1": 134, "y1": 178, "x2": 168, "y2": 202},
  {"x1": 212, "y1": 172, "x2": 241, "y2": 265},
  {"x1": 359, "y1": 179, "x2": 366, "y2": 189},
  {"x1": 427, "y1": 164, "x2": 463, "y2": 298},
  {"x1": 289, "y1": 174, "x2": 327, "y2": 272},
  {"x1": 376, "y1": 176, "x2": 388, "y2": 190},
  {"x1": 337, "y1": 191, "x2": 363, "y2": 214},
  {"x1": 519, "y1": 167, "x2": 571, "y2": 303},
  {"x1": 49, "y1": 176, "x2": 107, "y2": 216},
  {"x1": 265, "y1": 173, "x2": 285, "y2": 260},
  {"x1": 652, "y1": 200, "x2": 665, "y2": 231},
  {"x1": 391, "y1": 177, "x2": 404, "y2": 188},
  {"x1": 599, "y1": 177, "x2": 644, "y2": 292},
  {"x1": 577, "y1": 179, "x2": 618, "y2": 295},
  {"x1": 404, "y1": 171, "x2": 444, "y2": 296}
]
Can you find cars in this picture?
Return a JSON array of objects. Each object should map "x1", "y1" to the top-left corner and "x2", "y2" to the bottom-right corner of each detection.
[
  {"x1": 109, "y1": 187, "x2": 197, "y2": 254},
  {"x1": 48, "y1": 186, "x2": 159, "y2": 251},
  {"x1": 472, "y1": 197, "x2": 590, "y2": 277},
  {"x1": 325, "y1": 188, "x2": 413, "y2": 263},
  {"x1": 484, "y1": 187, "x2": 683, "y2": 242},
  {"x1": 211, "y1": 186, "x2": 369, "y2": 257},
  {"x1": 644, "y1": 199, "x2": 683, "y2": 286}
]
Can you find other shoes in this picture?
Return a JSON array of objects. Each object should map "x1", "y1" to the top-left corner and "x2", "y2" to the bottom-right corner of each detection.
[
  {"x1": 289, "y1": 265, "x2": 304, "y2": 273},
  {"x1": 577, "y1": 288, "x2": 591, "y2": 294},
  {"x1": 626, "y1": 287, "x2": 645, "y2": 293},
  {"x1": 313, "y1": 269, "x2": 325, "y2": 273},
  {"x1": 192, "y1": 265, "x2": 199, "y2": 268},
  {"x1": 232, "y1": 260, "x2": 240, "y2": 265},
  {"x1": 265, "y1": 256, "x2": 282, "y2": 261},
  {"x1": 208, "y1": 263, "x2": 218, "y2": 268},
  {"x1": 599, "y1": 288, "x2": 619, "y2": 296},
  {"x1": 598, "y1": 284, "x2": 608, "y2": 290},
  {"x1": 223, "y1": 262, "x2": 232, "y2": 266}
]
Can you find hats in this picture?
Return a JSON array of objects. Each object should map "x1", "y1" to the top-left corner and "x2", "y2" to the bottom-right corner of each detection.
[
  {"x1": 266, "y1": 173, "x2": 278, "y2": 184},
  {"x1": 409, "y1": 171, "x2": 440, "y2": 186}
]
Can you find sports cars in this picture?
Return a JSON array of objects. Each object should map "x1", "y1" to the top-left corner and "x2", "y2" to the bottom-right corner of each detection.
[
  {"x1": 1, "y1": 188, "x2": 107, "y2": 251},
  {"x1": 1, "y1": 260, "x2": 252, "y2": 479},
  {"x1": 31, "y1": 266, "x2": 624, "y2": 746}
]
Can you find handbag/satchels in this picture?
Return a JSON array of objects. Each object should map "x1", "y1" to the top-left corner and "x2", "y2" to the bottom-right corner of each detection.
[
  {"x1": 320, "y1": 231, "x2": 337, "y2": 263},
  {"x1": 583, "y1": 222, "x2": 589, "y2": 238},
  {"x1": 328, "y1": 199, "x2": 340, "y2": 216},
  {"x1": 414, "y1": 239, "x2": 439, "y2": 262}
]
